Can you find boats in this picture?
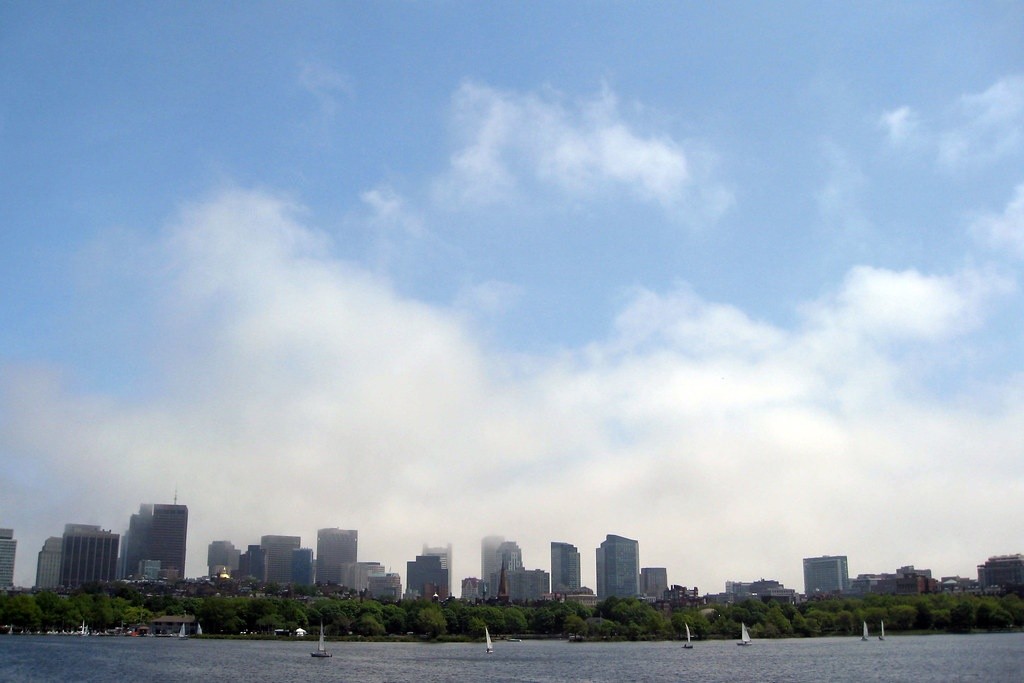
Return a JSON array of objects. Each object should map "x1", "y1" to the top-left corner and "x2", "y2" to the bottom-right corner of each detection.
[{"x1": 506, "y1": 637, "x2": 522, "y2": 642}]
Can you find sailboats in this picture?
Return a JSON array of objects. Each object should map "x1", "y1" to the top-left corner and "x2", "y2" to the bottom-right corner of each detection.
[
  {"x1": 736, "y1": 621, "x2": 753, "y2": 646},
  {"x1": 878, "y1": 619, "x2": 887, "y2": 640},
  {"x1": 178, "y1": 622, "x2": 189, "y2": 639},
  {"x1": 484, "y1": 627, "x2": 494, "y2": 653},
  {"x1": 682, "y1": 623, "x2": 693, "y2": 648},
  {"x1": 861, "y1": 620, "x2": 870, "y2": 642},
  {"x1": 311, "y1": 622, "x2": 332, "y2": 657}
]
